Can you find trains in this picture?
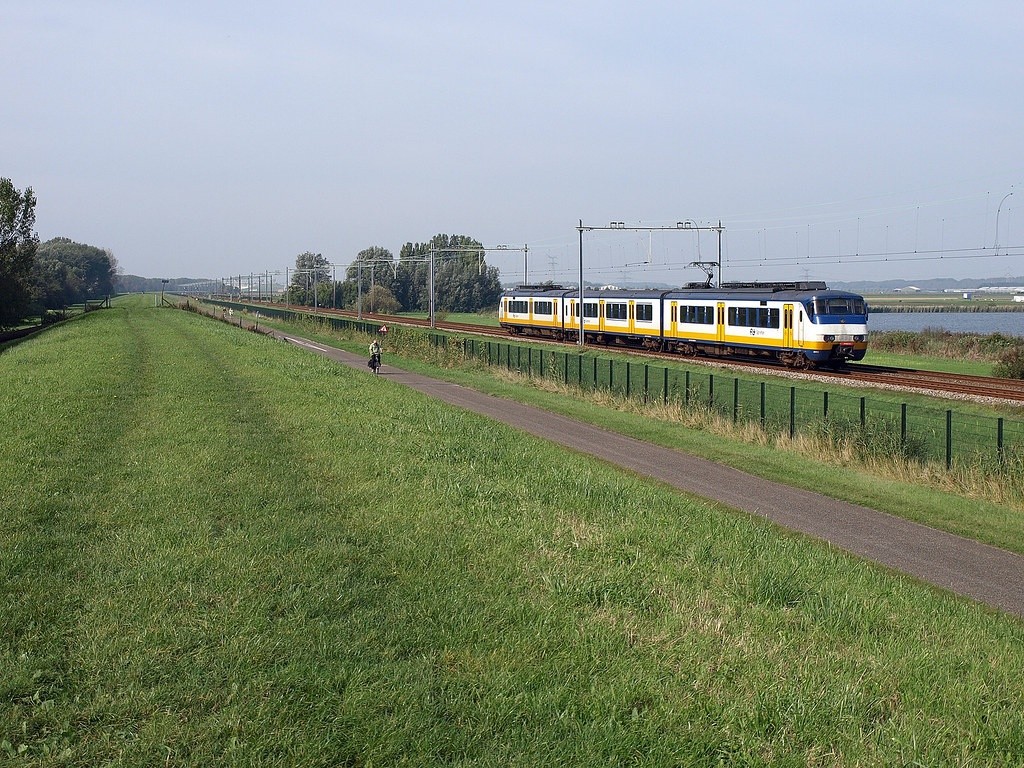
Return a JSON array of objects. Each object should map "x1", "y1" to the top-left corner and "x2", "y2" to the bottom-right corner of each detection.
[{"x1": 498, "y1": 280, "x2": 871, "y2": 372}]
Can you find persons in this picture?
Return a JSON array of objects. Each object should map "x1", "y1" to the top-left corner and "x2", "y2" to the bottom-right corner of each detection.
[{"x1": 368, "y1": 340, "x2": 382, "y2": 372}]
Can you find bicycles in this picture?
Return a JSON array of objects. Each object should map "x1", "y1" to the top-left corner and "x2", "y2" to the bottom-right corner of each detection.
[{"x1": 372, "y1": 352, "x2": 383, "y2": 375}]
